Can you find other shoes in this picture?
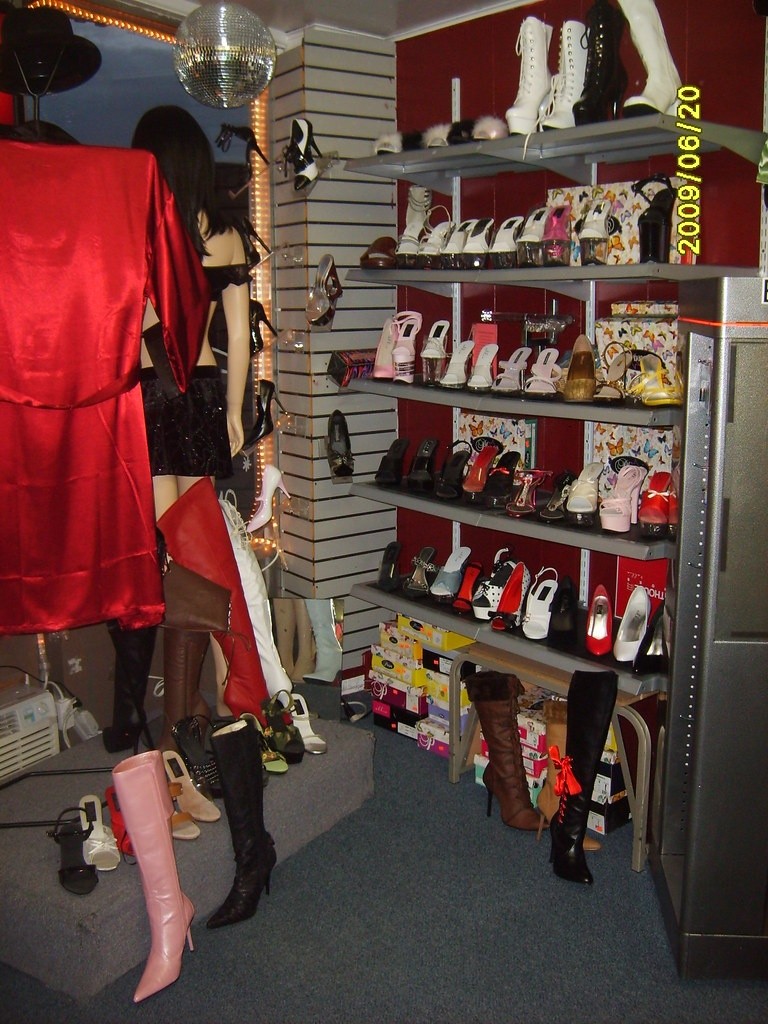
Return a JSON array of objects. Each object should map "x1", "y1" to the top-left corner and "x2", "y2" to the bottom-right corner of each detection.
[
  {"x1": 327, "y1": 409, "x2": 355, "y2": 475},
  {"x1": 373, "y1": 116, "x2": 507, "y2": 155}
]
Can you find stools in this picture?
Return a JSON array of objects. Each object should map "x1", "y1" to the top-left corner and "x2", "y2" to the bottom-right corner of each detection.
[{"x1": 449, "y1": 642, "x2": 666, "y2": 872}]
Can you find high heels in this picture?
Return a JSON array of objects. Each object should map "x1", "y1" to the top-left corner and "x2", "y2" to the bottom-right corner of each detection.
[
  {"x1": 359, "y1": 311, "x2": 676, "y2": 411},
  {"x1": 373, "y1": 430, "x2": 672, "y2": 534},
  {"x1": 378, "y1": 541, "x2": 670, "y2": 671},
  {"x1": 244, "y1": 693, "x2": 323, "y2": 787},
  {"x1": 240, "y1": 379, "x2": 287, "y2": 451},
  {"x1": 282, "y1": 118, "x2": 322, "y2": 192},
  {"x1": 357, "y1": 186, "x2": 675, "y2": 274},
  {"x1": 246, "y1": 463, "x2": 292, "y2": 532},
  {"x1": 216, "y1": 123, "x2": 269, "y2": 195},
  {"x1": 243, "y1": 217, "x2": 271, "y2": 270},
  {"x1": 157, "y1": 715, "x2": 220, "y2": 840},
  {"x1": 249, "y1": 299, "x2": 277, "y2": 354},
  {"x1": 50, "y1": 785, "x2": 135, "y2": 894},
  {"x1": 306, "y1": 255, "x2": 343, "y2": 324}
]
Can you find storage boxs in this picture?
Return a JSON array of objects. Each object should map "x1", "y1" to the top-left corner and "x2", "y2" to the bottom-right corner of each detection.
[
  {"x1": 525, "y1": 420, "x2": 537, "y2": 470},
  {"x1": 368, "y1": 611, "x2": 632, "y2": 836},
  {"x1": 458, "y1": 413, "x2": 525, "y2": 486},
  {"x1": 595, "y1": 317, "x2": 678, "y2": 395},
  {"x1": 611, "y1": 301, "x2": 679, "y2": 316},
  {"x1": 545, "y1": 177, "x2": 687, "y2": 268},
  {"x1": 592, "y1": 423, "x2": 682, "y2": 503}
]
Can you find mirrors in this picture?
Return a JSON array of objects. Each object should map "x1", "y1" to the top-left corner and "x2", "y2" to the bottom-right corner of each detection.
[{"x1": 269, "y1": 596, "x2": 344, "y2": 689}]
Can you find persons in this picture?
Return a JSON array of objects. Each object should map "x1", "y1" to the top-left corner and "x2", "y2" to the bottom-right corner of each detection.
[{"x1": 103, "y1": 103, "x2": 250, "y2": 753}]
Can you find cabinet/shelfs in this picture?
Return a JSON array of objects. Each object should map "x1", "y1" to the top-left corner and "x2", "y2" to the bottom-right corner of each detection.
[{"x1": 342, "y1": 115, "x2": 768, "y2": 695}]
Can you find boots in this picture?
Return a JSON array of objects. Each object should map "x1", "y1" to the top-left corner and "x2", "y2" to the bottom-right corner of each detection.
[
  {"x1": 500, "y1": 0, "x2": 692, "y2": 133},
  {"x1": 270, "y1": 596, "x2": 346, "y2": 687},
  {"x1": 101, "y1": 614, "x2": 157, "y2": 754},
  {"x1": 465, "y1": 671, "x2": 542, "y2": 830},
  {"x1": 205, "y1": 721, "x2": 276, "y2": 929},
  {"x1": 154, "y1": 565, "x2": 230, "y2": 746},
  {"x1": 549, "y1": 670, "x2": 624, "y2": 886},
  {"x1": 160, "y1": 481, "x2": 300, "y2": 725},
  {"x1": 108, "y1": 751, "x2": 195, "y2": 1003}
]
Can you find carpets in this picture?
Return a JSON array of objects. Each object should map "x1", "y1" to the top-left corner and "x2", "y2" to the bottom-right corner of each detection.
[{"x1": 1, "y1": 689, "x2": 374, "y2": 1010}]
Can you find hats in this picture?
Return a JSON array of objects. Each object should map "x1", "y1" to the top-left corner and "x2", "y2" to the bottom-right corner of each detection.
[{"x1": 0, "y1": 7, "x2": 104, "y2": 98}]
